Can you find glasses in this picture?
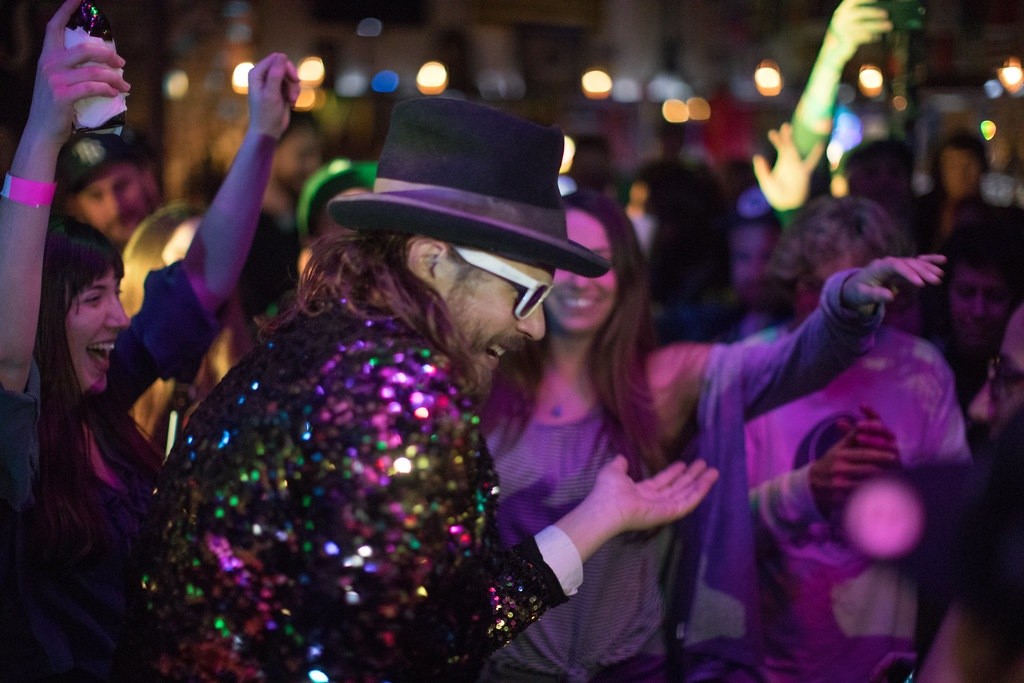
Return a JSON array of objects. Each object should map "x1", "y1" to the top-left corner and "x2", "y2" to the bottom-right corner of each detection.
[{"x1": 455, "y1": 247, "x2": 555, "y2": 321}]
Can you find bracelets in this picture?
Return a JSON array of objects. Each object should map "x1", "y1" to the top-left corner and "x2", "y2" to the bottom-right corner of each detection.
[{"x1": 1, "y1": 174, "x2": 57, "y2": 208}]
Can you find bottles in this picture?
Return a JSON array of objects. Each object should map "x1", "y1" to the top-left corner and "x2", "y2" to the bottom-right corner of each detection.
[{"x1": 61, "y1": 1, "x2": 125, "y2": 133}]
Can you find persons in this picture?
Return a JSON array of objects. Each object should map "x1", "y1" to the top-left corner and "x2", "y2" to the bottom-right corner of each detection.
[{"x1": 0, "y1": 0, "x2": 1024, "y2": 683}]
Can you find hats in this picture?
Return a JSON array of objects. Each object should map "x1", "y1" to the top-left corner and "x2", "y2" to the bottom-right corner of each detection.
[
  {"x1": 53, "y1": 123, "x2": 146, "y2": 196},
  {"x1": 326, "y1": 95, "x2": 611, "y2": 279}
]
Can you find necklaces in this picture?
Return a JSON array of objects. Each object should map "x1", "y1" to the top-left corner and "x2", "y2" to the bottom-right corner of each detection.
[{"x1": 543, "y1": 387, "x2": 569, "y2": 416}]
